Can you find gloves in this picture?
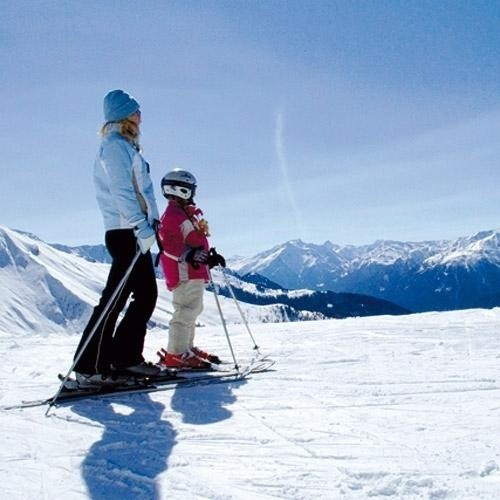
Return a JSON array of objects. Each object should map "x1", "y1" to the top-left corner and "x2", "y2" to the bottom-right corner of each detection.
[
  {"x1": 187, "y1": 248, "x2": 208, "y2": 263},
  {"x1": 133, "y1": 221, "x2": 157, "y2": 254}
]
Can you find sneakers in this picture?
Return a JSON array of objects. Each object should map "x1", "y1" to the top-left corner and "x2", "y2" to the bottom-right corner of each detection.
[
  {"x1": 165, "y1": 349, "x2": 204, "y2": 370},
  {"x1": 75, "y1": 371, "x2": 130, "y2": 389},
  {"x1": 191, "y1": 347, "x2": 209, "y2": 363},
  {"x1": 126, "y1": 361, "x2": 161, "y2": 376}
]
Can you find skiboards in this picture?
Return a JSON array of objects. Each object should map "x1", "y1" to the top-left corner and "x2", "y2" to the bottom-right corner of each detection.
[{"x1": 2, "y1": 352, "x2": 276, "y2": 410}]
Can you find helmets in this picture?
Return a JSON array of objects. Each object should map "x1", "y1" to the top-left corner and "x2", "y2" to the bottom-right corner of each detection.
[{"x1": 161, "y1": 168, "x2": 197, "y2": 201}]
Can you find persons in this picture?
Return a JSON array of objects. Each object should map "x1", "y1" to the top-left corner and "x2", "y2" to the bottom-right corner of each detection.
[
  {"x1": 157, "y1": 171, "x2": 219, "y2": 369},
  {"x1": 73, "y1": 90, "x2": 158, "y2": 385}
]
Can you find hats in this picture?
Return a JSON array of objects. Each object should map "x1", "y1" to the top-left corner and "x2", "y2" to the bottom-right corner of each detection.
[{"x1": 103, "y1": 89, "x2": 140, "y2": 122}]
